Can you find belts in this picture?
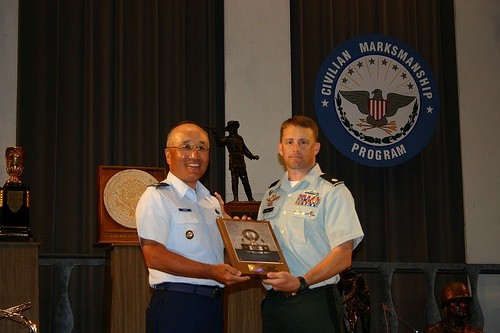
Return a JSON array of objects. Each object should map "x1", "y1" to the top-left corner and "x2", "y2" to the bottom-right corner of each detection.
[{"x1": 156, "y1": 281, "x2": 222, "y2": 298}]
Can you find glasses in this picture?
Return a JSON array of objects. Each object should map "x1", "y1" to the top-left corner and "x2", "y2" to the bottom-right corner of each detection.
[{"x1": 166, "y1": 144, "x2": 210, "y2": 155}]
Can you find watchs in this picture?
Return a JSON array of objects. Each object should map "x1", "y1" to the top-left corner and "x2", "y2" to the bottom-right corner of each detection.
[{"x1": 296, "y1": 277, "x2": 309, "y2": 293}]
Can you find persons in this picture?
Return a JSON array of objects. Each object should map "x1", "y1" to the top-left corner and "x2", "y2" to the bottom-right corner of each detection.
[
  {"x1": 135, "y1": 122, "x2": 251, "y2": 333},
  {"x1": 211, "y1": 121, "x2": 260, "y2": 201},
  {"x1": 215, "y1": 115, "x2": 364, "y2": 333},
  {"x1": 423, "y1": 281, "x2": 484, "y2": 333}
]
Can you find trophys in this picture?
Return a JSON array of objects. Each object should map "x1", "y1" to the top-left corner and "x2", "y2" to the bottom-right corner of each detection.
[{"x1": 0, "y1": 146, "x2": 31, "y2": 242}]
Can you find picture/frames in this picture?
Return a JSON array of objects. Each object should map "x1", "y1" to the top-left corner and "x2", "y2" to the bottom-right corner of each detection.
[{"x1": 218, "y1": 216, "x2": 291, "y2": 278}]
